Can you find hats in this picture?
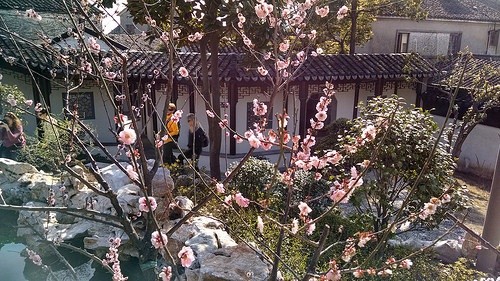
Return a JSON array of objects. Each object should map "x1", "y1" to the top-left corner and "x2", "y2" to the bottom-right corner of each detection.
[{"x1": 183, "y1": 113, "x2": 195, "y2": 121}]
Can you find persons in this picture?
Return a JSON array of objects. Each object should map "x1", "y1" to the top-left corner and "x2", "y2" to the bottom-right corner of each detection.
[
  {"x1": 156, "y1": 102, "x2": 180, "y2": 166},
  {"x1": 0, "y1": 113, "x2": 26, "y2": 161},
  {"x1": 178, "y1": 112, "x2": 209, "y2": 180},
  {"x1": 36, "y1": 107, "x2": 61, "y2": 150}
]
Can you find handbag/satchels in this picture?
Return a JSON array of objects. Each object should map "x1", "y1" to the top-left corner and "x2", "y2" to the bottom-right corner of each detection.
[{"x1": 201, "y1": 133, "x2": 209, "y2": 149}]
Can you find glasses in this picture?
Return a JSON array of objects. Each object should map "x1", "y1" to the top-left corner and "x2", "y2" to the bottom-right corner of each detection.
[
  {"x1": 188, "y1": 119, "x2": 192, "y2": 123},
  {"x1": 38, "y1": 113, "x2": 45, "y2": 117},
  {"x1": 169, "y1": 109, "x2": 174, "y2": 112}
]
